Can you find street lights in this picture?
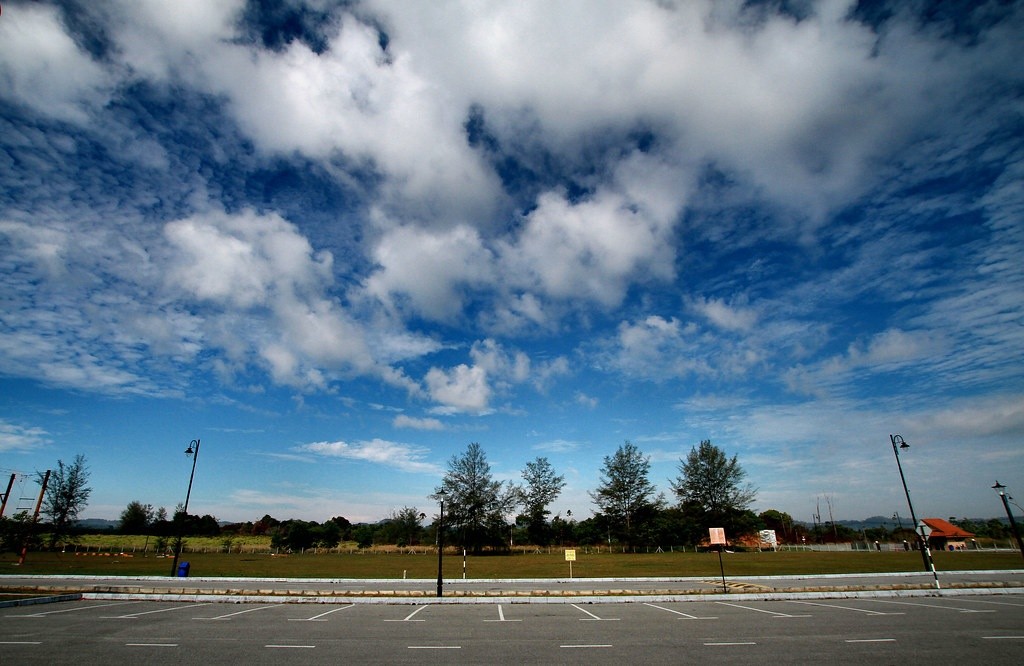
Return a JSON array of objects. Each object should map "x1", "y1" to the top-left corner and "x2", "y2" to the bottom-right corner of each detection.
[
  {"x1": 991, "y1": 479, "x2": 1024, "y2": 561},
  {"x1": 170, "y1": 438, "x2": 200, "y2": 577},
  {"x1": 435, "y1": 486, "x2": 448, "y2": 596},
  {"x1": 889, "y1": 433, "x2": 931, "y2": 572}
]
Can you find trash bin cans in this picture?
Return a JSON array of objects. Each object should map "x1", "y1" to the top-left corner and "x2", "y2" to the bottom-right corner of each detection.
[{"x1": 177, "y1": 562, "x2": 190, "y2": 577}]
